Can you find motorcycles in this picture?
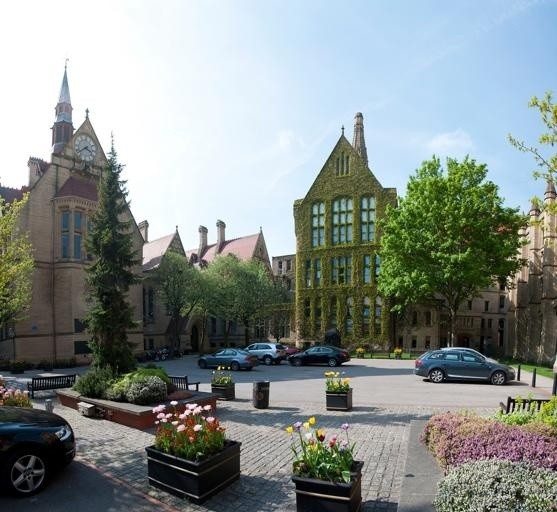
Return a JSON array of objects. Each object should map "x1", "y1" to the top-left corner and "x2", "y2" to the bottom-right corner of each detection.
[{"x1": 145, "y1": 343, "x2": 184, "y2": 362}]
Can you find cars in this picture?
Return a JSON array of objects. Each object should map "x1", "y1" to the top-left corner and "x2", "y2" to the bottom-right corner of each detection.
[
  {"x1": 0, "y1": 405, "x2": 76, "y2": 496},
  {"x1": 197, "y1": 342, "x2": 350, "y2": 372},
  {"x1": 415, "y1": 347, "x2": 515, "y2": 385}
]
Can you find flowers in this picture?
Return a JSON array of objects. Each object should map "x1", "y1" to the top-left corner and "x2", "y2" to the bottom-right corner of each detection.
[
  {"x1": 355, "y1": 348, "x2": 366, "y2": 353},
  {"x1": 208, "y1": 365, "x2": 232, "y2": 386},
  {"x1": 151, "y1": 400, "x2": 226, "y2": 460},
  {"x1": 0, "y1": 374, "x2": 33, "y2": 409},
  {"x1": 287, "y1": 416, "x2": 358, "y2": 483},
  {"x1": 394, "y1": 346, "x2": 403, "y2": 354},
  {"x1": 324, "y1": 370, "x2": 352, "y2": 394}
]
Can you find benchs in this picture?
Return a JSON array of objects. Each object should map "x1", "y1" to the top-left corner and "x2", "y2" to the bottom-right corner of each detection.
[
  {"x1": 168, "y1": 375, "x2": 201, "y2": 391},
  {"x1": 27, "y1": 373, "x2": 76, "y2": 399},
  {"x1": 500, "y1": 396, "x2": 550, "y2": 414}
]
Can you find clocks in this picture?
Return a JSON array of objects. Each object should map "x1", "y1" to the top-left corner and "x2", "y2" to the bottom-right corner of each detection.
[{"x1": 74, "y1": 133, "x2": 98, "y2": 161}]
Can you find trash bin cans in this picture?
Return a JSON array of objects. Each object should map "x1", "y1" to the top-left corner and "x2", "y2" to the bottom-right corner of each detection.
[{"x1": 253, "y1": 381, "x2": 270, "y2": 409}]
